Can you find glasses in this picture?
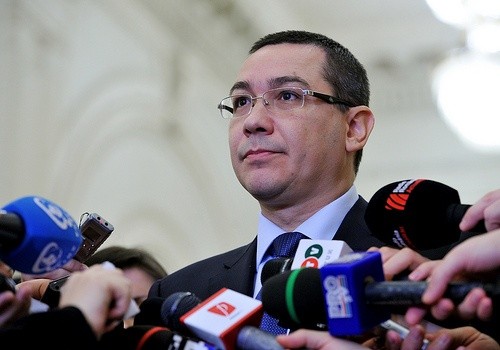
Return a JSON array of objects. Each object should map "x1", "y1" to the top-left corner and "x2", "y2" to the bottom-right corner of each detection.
[{"x1": 218, "y1": 86, "x2": 357, "y2": 119}]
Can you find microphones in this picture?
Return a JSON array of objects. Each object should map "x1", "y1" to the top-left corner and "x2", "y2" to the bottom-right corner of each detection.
[
  {"x1": 113, "y1": 179, "x2": 500, "y2": 350},
  {"x1": 0, "y1": 195, "x2": 82, "y2": 275}
]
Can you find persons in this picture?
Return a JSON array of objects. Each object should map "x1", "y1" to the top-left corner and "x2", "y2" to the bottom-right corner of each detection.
[
  {"x1": 0, "y1": 188, "x2": 500, "y2": 350},
  {"x1": 146, "y1": 29, "x2": 448, "y2": 335}
]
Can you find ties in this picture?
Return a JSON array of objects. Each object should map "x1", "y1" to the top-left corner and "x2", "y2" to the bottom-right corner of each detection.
[{"x1": 250, "y1": 232, "x2": 312, "y2": 335}]
